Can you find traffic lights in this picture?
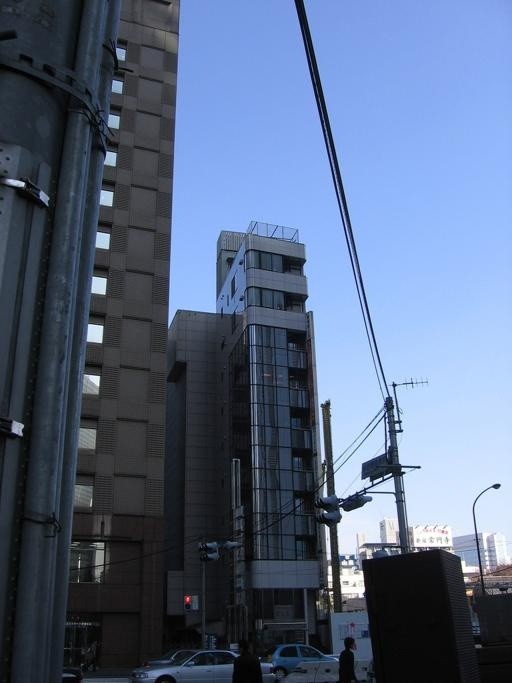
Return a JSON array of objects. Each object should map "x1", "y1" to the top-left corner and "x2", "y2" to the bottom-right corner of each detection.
[
  {"x1": 199, "y1": 542, "x2": 219, "y2": 562},
  {"x1": 184, "y1": 595, "x2": 192, "y2": 612},
  {"x1": 315, "y1": 494, "x2": 342, "y2": 526}
]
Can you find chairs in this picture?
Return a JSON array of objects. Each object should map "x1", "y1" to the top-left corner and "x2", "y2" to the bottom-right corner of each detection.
[{"x1": 204, "y1": 656, "x2": 218, "y2": 665}]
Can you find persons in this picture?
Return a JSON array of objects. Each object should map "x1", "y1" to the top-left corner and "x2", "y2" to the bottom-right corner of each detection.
[
  {"x1": 337, "y1": 635, "x2": 360, "y2": 683},
  {"x1": 84, "y1": 638, "x2": 100, "y2": 671},
  {"x1": 230, "y1": 638, "x2": 264, "y2": 682}
]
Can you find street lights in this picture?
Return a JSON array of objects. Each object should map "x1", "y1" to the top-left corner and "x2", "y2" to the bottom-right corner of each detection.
[{"x1": 473, "y1": 483, "x2": 501, "y2": 596}]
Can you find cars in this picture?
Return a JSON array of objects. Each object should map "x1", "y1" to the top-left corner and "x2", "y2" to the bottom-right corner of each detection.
[
  {"x1": 128, "y1": 644, "x2": 340, "y2": 683},
  {"x1": 61, "y1": 669, "x2": 82, "y2": 682}
]
